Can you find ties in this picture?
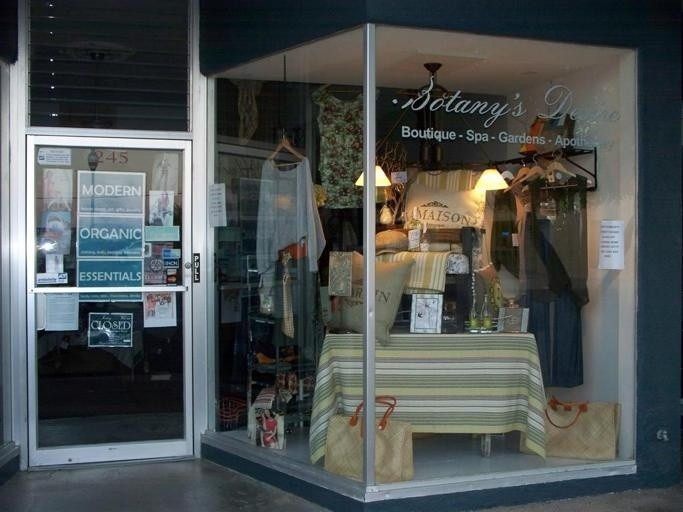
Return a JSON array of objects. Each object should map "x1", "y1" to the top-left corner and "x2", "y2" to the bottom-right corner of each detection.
[{"x1": 282, "y1": 253, "x2": 294, "y2": 339}]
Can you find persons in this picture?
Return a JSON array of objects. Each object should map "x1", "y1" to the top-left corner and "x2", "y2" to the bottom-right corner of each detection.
[
  {"x1": 154, "y1": 191, "x2": 171, "y2": 221},
  {"x1": 154, "y1": 153, "x2": 172, "y2": 191},
  {"x1": 43, "y1": 170, "x2": 70, "y2": 211}
]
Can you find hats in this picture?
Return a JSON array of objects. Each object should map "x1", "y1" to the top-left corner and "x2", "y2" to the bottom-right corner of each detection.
[{"x1": 376, "y1": 230, "x2": 408, "y2": 256}]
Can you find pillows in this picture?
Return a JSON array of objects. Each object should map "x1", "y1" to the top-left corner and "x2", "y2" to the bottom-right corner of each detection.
[{"x1": 327, "y1": 251, "x2": 415, "y2": 346}]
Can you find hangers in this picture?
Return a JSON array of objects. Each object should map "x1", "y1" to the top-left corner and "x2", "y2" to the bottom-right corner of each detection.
[
  {"x1": 269, "y1": 127, "x2": 305, "y2": 168},
  {"x1": 503, "y1": 149, "x2": 592, "y2": 194},
  {"x1": 323, "y1": 82, "x2": 363, "y2": 93}
]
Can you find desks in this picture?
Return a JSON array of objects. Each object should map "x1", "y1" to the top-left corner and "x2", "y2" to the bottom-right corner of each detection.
[{"x1": 324, "y1": 322, "x2": 535, "y2": 458}]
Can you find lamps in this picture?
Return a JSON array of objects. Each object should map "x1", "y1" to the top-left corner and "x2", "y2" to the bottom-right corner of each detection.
[{"x1": 354, "y1": 64, "x2": 509, "y2": 191}]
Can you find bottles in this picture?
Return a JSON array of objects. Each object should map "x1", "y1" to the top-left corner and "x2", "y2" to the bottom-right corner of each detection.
[{"x1": 469, "y1": 292, "x2": 493, "y2": 334}]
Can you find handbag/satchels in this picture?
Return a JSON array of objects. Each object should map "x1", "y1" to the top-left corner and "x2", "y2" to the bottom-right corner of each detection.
[
  {"x1": 520, "y1": 394, "x2": 621, "y2": 461},
  {"x1": 518, "y1": 113, "x2": 575, "y2": 154},
  {"x1": 325, "y1": 395, "x2": 413, "y2": 484}
]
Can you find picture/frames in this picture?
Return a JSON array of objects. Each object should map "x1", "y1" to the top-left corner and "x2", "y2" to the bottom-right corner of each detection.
[{"x1": 409, "y1": 293, "x2": 444, "y2": 334}]
